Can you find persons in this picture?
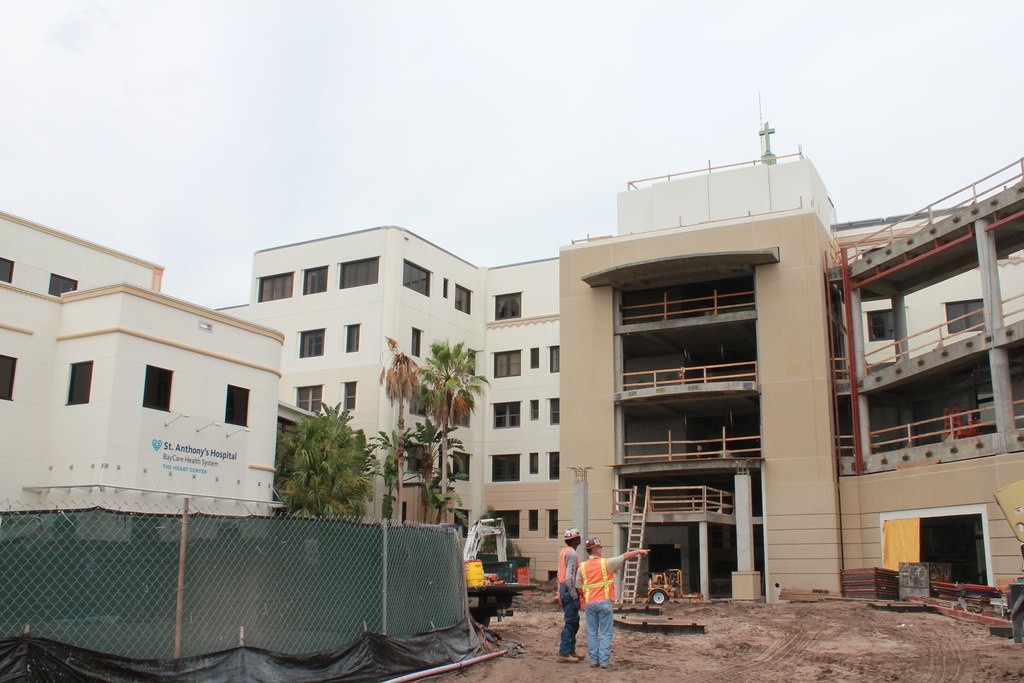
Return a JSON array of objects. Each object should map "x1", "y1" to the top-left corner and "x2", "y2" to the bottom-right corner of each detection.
[
  {"x1": 556, "y1": 528, "x2": 586, "y2": 664},
  {"x1": 574, "y1": 537, "x2": 651, "y2": 668}
]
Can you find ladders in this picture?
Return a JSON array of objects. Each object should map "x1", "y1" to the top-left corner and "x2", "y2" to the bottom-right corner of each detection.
[{"x1": 619, "y1": 486, "x2": 649, "y2": 605}]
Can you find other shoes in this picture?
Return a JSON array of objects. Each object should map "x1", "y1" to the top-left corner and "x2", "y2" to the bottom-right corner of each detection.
[{"x1": 590, "y1": 662, "x2": 615, "y2": 668}]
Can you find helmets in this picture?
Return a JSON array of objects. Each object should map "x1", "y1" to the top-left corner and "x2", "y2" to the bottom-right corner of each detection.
[
  {"x1": 564, "y1": 527, "x2": 580, "y2": 540},
  {"x1": 584, "y1": 537, "x2": 603, "y2": 550}
]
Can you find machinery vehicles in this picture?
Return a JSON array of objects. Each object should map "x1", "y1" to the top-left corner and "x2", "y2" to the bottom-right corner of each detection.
[
  {"x1": 642, "y1": 568, "x2": 703, "y2": 605},
  {"x1": 439, "y1": 517, "x2": 538, "y2": 627}
]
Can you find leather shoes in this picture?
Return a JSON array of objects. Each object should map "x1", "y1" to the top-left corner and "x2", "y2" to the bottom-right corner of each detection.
[{"x1": 557, "y1": 652, "x2": 585, "y2": 662}]
